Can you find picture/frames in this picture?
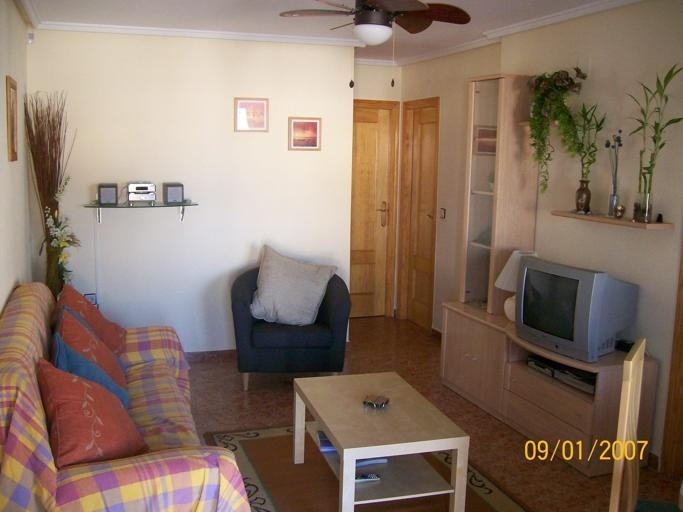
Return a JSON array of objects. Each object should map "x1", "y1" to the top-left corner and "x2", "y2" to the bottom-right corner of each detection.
[
  {"x1": 4, "y1": 76, "x2": 19, "y2": 163},
  {"x1": 289, "y1": 117, "x2": 321, "y2": 151},
  {"x1": 233, "y1": 97, "x2": 268, "y2": 132},
  {"x1": 473, "y1": 125, "x2": 499, "y2": 157}
]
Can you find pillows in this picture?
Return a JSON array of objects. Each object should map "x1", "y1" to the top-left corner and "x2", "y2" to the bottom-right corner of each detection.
[
  {"x1": 250, "y1": 246, "x2": 338, "y2": 327},
  {"x1": 34, "y1": 284, "x2": 148, "y2": 468}
]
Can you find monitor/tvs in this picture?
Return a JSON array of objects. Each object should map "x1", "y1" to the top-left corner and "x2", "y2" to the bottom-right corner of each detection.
[{"x1": 515, "y1": 256, "x2": 640, "y2": 364}]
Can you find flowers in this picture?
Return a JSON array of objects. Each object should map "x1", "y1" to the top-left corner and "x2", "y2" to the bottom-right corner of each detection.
[{"x1": 37, "y1": 174, "x2": 78, "y2": 293}]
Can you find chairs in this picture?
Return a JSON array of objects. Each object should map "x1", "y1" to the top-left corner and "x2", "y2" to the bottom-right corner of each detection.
[
  {"x1": 231, "y1": 267, "x2": 352, "y2": 390},
  {"x1": 608, "y1": 334, "x2": 681, "y2": 511}
]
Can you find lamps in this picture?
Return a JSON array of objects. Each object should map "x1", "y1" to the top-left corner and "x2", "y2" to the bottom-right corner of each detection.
[
  {"x1": 495, "y1": 248, "x2": 538, "y2": 322},
  {"x1": 350, "y1": 24, "x2": 396, "y2": 45}
]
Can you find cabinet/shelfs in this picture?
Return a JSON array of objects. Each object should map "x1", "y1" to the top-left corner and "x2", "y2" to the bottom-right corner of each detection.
[
  {"x1": 438, "y1": 299, "x2": 661, "y2": 476},
  {"x1": 457, "y1": 75, "x2": 538, "y2": 315}
]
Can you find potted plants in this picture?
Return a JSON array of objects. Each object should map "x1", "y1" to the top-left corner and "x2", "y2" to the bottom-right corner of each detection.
[
  {"x1": 531, "y1": 66, "x2": 606, "y2": 219},
  {"x1": 627, "y1": 64, "x2": 681, "y2": 223},
  {"x1": 603, "y1": 131, "x2": 627, "y2": 219}
]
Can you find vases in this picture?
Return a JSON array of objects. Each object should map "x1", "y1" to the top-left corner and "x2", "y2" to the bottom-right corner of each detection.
[{"x1": 44, "y1": 244, "x2": 64, "y2": 296}]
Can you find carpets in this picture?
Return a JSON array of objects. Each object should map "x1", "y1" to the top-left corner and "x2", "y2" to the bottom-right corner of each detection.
[{"x1": 202, "y1": 420, "x2": 532, "y2": 510}]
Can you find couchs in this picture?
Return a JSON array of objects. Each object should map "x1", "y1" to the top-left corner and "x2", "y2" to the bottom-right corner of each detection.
[{"x1": 1, "y1": 282, "x2": 250, "y2": 512}]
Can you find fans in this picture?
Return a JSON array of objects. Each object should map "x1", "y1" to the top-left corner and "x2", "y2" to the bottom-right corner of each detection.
[{"x1": 278, "y1": 0, "x2": 472, "y2": 35}]
